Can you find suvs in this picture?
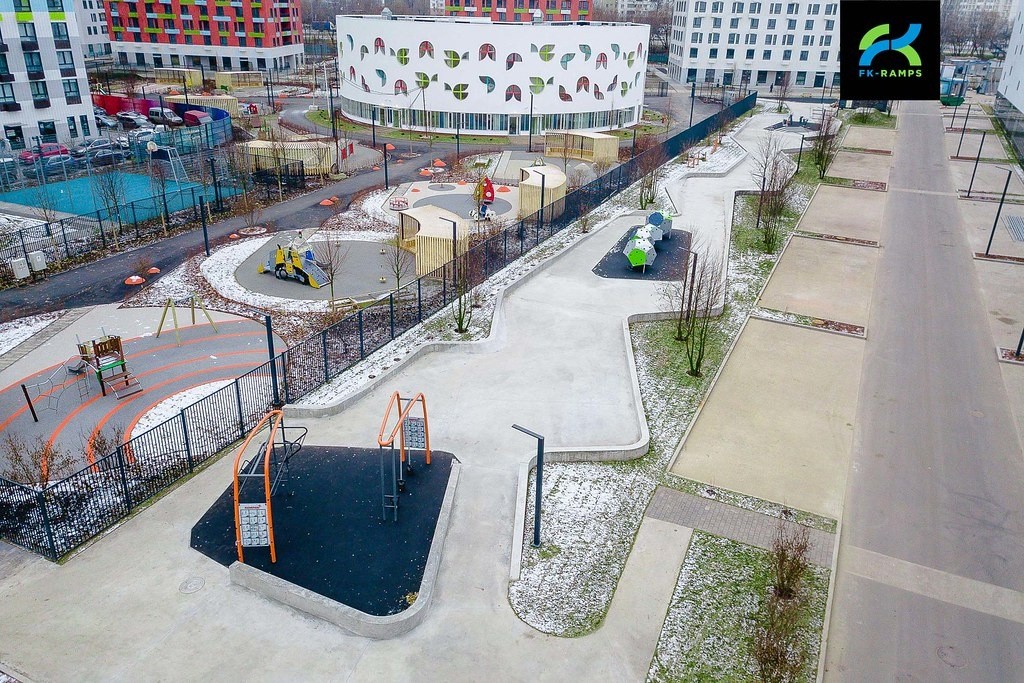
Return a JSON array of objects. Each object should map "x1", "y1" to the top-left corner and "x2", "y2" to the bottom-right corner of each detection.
[
  {"x1": 116, "y1": 128, "x2": 154, "y2": 149},
  {"x1": 0, "y1": 154, "x2": 17, "y2": 185},
  {"x1": 22, "y1": 154, "x2": 77, "y2": 179}
]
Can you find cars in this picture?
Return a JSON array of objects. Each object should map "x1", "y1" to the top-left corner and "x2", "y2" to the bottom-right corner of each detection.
[
  {"x1": 19, "y1": 142, "x2": 69, "y2": 167},
  {"x1": 92, "y1": 103, "x2": 173, "y2": 138},
  {"x1": 75, "y1": 148, "x2": 123, "y2": 170},
  {"x1": 71, "y1": 138, "x2": 116, "y2": 158},
  {"x1": 123, "y1": 140, "x2": 152, "y2": 159}
]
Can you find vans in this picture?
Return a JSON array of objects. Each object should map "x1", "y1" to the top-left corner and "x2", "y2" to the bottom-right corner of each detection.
[
  {"x1": 184, "y1": 110, "x2": 214, "y2": 128},
  {"x1": 149, "y1": 107, "x2": 184, "y2": 126}
]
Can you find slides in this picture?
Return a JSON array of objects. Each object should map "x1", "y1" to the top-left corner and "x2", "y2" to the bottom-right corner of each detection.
[{"x1": 68, "y1": 360, "x2": 84, "y2": 370}]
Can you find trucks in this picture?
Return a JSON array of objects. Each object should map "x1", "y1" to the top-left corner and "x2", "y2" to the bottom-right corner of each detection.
[{"x1": 313, "y1": 21, "x2": 334, "y2": 32}]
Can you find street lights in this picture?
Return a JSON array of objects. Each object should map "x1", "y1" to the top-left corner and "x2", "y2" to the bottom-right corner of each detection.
[
  {"x1": 247, "y1": 307, "x2": 281, "y2": 407},
  {"x1": 534, "y1": 169, "x2": 545, "y2": 230},
  {"x1": 199, "y1": 193, "x2": 215, "y2": 258},
  {"x1": 794, "y1": 131, "x2": 805, "y2": 173},
  {"x1": 457, "y1": 122, "x2": 469, "y2": 157},
  {"x1": 950, "y1": 72, "x2": 987, "y2": 198},
  {"x1": 440, "y1": 215, "x2": 458, "y2": 290},
  {"x1": 984, "y1": 164, "x2": 1014, "y2": 257},
  {"x1": 677, "y1": 246, "x2": 699, "y2": 325},
  {"x1": 754, "y1": 172, "x2": 766, "y2": 229},
  {"x1": 511, "y1": 424, "x2": 546, "y2": 548},
  {"x1": 627, "y1": 127, "x2": 637, "y2": 159},
  {"x1": 529, "y1": 92, "x2": 533, "y2": 152}
]
